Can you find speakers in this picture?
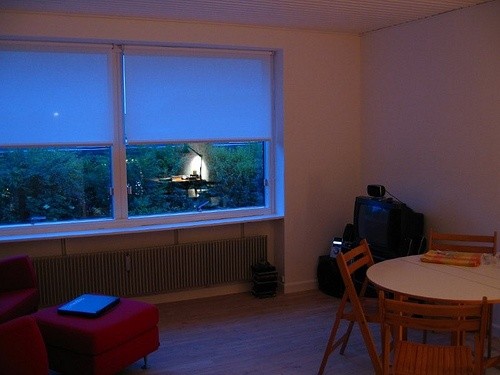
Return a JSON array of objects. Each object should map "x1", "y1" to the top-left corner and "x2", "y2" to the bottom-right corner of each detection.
[
  {"x1": 343, "y1": 224, "x2": 354, "y2": 243},
  {"x1": 353, "y1": 196, "x2": 424, "y2": 259},
  {"x1": 366, "y1": 185, "x2": 386, "y2": 197}
]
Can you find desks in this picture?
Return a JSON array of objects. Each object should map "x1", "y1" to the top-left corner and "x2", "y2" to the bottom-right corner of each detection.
[
  {"x1": 367, "y1": 252, "x2": 500, "y2": 375},
  {"x1": 148, "y1": 177, "x2": 219, "y2": 211}
]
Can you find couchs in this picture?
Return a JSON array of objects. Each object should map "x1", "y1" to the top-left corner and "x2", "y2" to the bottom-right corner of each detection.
[{"x1": 0, "y1": 254, "x2": 49, "y2": 375}]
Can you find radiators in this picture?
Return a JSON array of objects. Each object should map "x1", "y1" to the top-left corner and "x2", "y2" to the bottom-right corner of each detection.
[{"x1": 32, "y1": 235, "x2": 268, "y2": 308}]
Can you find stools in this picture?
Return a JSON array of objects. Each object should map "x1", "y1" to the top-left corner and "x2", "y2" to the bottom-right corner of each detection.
[{"x1": 32, "y1": 296, "x2": 161, "y2": 375}]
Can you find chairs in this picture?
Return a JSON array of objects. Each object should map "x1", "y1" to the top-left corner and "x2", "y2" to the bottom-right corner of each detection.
[
  {"x1": 316, "y1": 239, "x2": 427, "y2": 375},
  {"x1": 378, "y1": 290, "x2": 488, "y2": 375},
  {"x1": 421, "y1": 228, "x2": 497, "y2": 348}
]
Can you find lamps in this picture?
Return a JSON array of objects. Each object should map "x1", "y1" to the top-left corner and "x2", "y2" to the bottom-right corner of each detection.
[{"x1": 180, "y1": 143, "x2": 203, "y2": 181}]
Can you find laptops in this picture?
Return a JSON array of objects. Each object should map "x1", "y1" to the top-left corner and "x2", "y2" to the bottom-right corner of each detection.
[{"x1": 57, "y1": 294, "x2": 121, "y2": 317}]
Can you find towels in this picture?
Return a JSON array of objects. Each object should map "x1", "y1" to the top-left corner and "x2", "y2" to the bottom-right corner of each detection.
[{"x1": 419, "y1": 250, "x2": 482, "y2": 267}]
